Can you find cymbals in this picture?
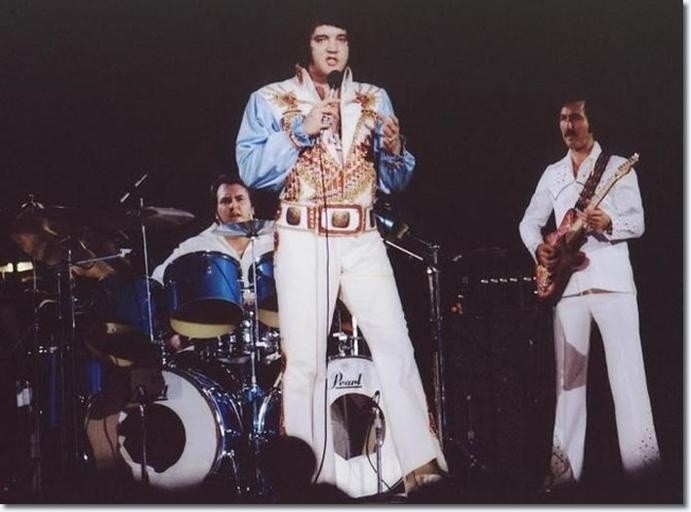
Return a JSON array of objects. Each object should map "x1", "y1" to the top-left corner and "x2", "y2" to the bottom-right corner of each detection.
[
  {"x1": 127, "y1": 207, "x2": 194, "y2": 225},
  {"x1": 213, "y1": 219, "x2": 275, "y2": 237}
]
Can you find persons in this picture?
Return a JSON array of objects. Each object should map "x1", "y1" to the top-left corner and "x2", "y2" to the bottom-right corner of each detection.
[
  {"x1": 233, "y1": 9, "x2": 450, "y2": 496},
  {"x1": 147, "y1": 175, "x2": 278, "y2": 287},
  {"x1": 515, "y1": 90, "x2": 661, "y2": 487}
]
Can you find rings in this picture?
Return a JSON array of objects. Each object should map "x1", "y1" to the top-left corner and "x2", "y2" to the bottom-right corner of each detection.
[{"x1": 321, "y1": 114, "x2": 331, "y2": 123}]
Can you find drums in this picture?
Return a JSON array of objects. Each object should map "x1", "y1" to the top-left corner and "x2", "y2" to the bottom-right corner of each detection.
[
  {"x1": 203, "y1": 298, "x2": 282, "y2": 365},
  {"x1": 84, "y1": 366, "x2": 246, "y2": 493},
  {"x1": 256, "y1": 354, "x2": 402, "y2": 499},
  {"x1": 163, "y1": 251, "x2": 242, "y2": 339},
  {"x1": 249, "y1": 251, "x2": 278, "y2": 330}
]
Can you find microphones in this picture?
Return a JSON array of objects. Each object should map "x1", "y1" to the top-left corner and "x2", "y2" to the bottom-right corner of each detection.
[
  {"x1": 118, "y1": 169, "x2": 149, "y2": 203},
  {"x1": 321, "y1": 69, "x2": 343, "y2": 135}
]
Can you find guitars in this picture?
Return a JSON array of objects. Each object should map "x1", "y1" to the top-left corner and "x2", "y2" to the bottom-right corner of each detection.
[{"x1": 536, "y1": 153, "x2": 639, "y2": 306}]
